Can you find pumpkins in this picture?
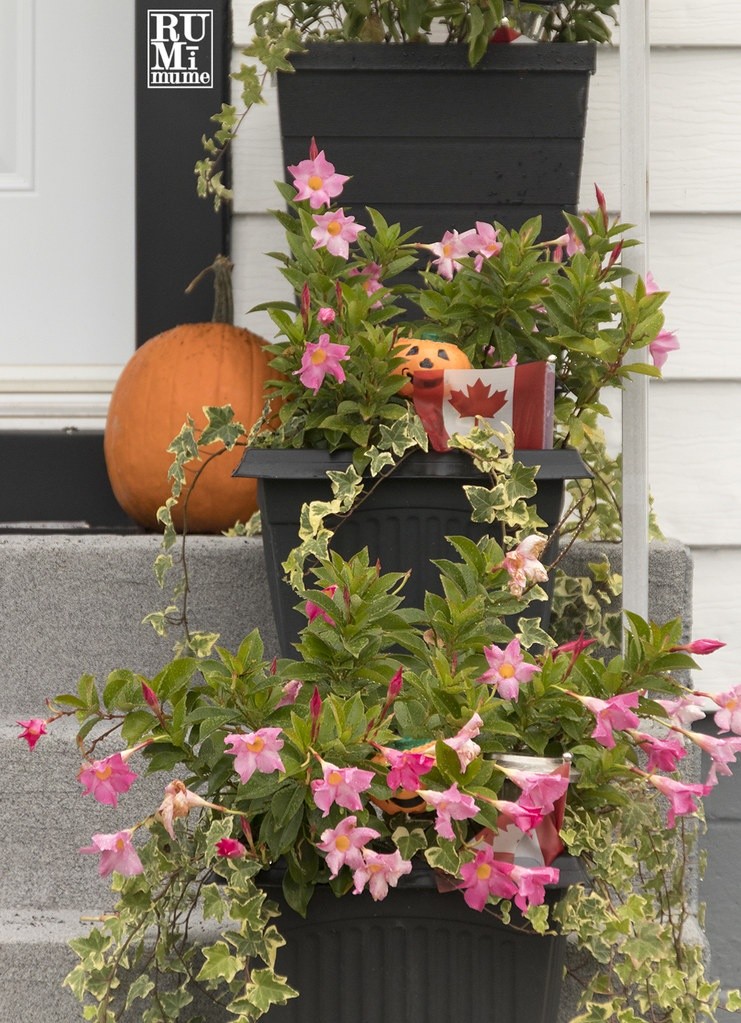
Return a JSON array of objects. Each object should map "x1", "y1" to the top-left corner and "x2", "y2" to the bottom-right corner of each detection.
[
  {"x1": 104, "y1": 255, "x2": 302, "y2": 534},
  {"x1": 389, "y1": 337, "x2": 472, "y2": 398}
]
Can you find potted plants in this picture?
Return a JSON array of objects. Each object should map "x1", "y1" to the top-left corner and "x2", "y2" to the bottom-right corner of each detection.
[{"x1": 190, "y1": 0, "x2": 621, "y2": 335}]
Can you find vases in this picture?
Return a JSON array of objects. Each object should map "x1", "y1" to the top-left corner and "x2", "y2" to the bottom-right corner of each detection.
[
  {"x1": 198, "y1": 846, "x2": 594, "y2": 1023},
  {"x1": 229, "y1": 445, "x2": 598, "y2": 666}
]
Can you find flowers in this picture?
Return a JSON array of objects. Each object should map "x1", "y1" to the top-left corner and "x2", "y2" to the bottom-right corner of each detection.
[
  {"x1": 244, "y1": 134, "x2": 685, "y2": 452},
  {"x1": 17, "y1": 526, "x2": 741, "y2": 1022}
]
